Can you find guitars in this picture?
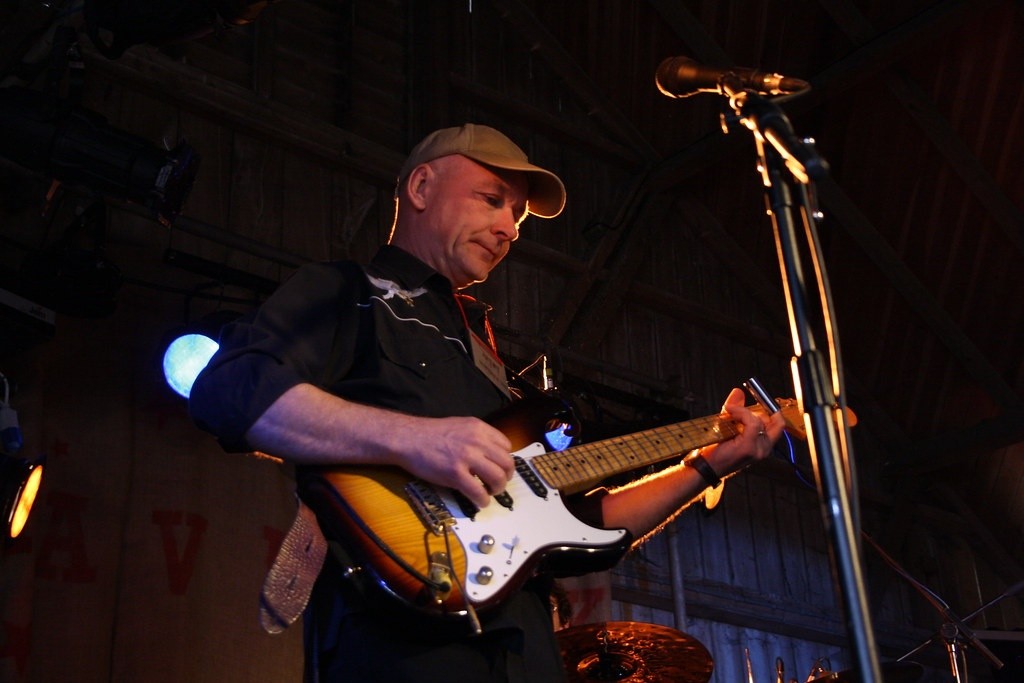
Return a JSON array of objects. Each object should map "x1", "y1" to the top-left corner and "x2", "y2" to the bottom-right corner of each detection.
[{"x1": 305, "y1": 394, "x2": 858, "y2": 614}]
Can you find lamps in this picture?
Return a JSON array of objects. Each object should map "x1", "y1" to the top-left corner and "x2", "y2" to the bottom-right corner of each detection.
[
  {"x1": 162, "y1": 329, "x2": 220, "y2": 398},
  {"x1": 0, "y1": 453, "x2": 43, "y2": 540}
]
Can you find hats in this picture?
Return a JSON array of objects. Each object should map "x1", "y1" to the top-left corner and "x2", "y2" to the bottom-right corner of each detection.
[{"x1": 397, "y1": 123, "x2": 566, "y2": 219}]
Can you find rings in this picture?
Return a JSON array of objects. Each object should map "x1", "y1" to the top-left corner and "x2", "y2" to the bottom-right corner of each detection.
[{"x1": 759, "y1": 429, "x2": 766, "y2": 436}]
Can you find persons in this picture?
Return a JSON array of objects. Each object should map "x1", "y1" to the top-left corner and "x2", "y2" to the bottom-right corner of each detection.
[{"x1": 187, "y1": 117, "x2": 789, "y2": 681}]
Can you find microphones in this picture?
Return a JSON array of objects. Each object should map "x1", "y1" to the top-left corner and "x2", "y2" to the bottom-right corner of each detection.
[{"x1": 655, "y1": 58, "x2": 807, "y2": 106}]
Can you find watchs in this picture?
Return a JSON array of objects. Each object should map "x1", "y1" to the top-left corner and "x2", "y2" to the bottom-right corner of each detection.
[{"x1": 681, "y1": 447, "x2": 723, "y2": 490}]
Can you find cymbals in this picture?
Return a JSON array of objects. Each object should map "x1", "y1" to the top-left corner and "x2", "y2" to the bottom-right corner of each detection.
[
  {"x1": 801, "y1": 661, "x2": 924, "y2": 683},
  {"x1": 552, "y1": 622, "x2": 716, "y2": 683}
]
